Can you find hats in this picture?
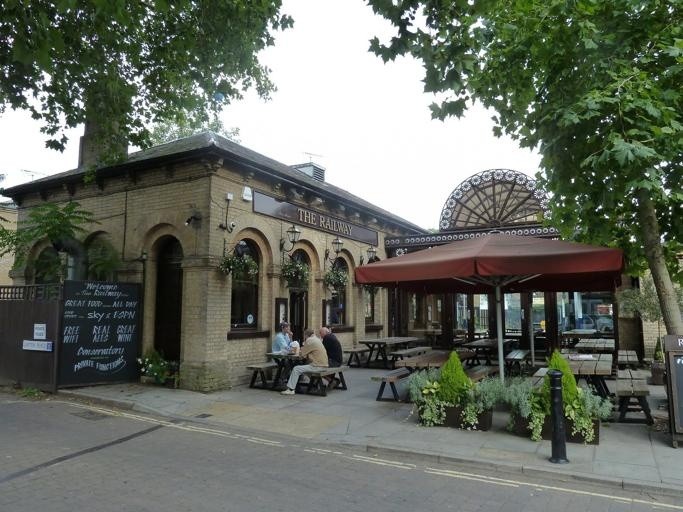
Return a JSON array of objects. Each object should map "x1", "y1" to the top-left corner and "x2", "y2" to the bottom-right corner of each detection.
[{"x1": 280, "y1": 322, "x2": 289, "y2": 328}]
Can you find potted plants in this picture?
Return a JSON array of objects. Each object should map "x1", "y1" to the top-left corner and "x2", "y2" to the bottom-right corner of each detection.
[{"x1": 650, "y1": 337, "x2": 666, "y2": 384}]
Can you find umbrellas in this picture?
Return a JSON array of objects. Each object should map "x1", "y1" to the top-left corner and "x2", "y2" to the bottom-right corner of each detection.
[{"x1": 354, "y1": 231, "x2": 625, "y2": 404}]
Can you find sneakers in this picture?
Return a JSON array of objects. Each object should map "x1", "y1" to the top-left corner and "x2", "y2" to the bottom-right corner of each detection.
[{"x1": 280, "y1": 389, "x2": 295, "y2": 395}]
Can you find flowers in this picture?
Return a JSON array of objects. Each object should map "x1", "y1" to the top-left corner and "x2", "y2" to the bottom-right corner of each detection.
[
  {"x1": 323, "y1": 268, "x2": 348, "y2": 285},
  {"x1": 157, "y1": 357, "x2": 178, "y2": 383},
  {"x1": 136, "y1": 347, "x2": 160, "y2": 376},
  {"x1": 280, "y1": 261, "x2": 309, "y2": 281},
  {"x1": 221, "y1": 254, "x2": 258, "y2": 277}
]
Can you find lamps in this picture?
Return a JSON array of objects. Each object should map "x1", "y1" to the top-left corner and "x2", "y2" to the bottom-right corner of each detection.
[
  {"x1": 280, "y1": 223, "x2": 301, "y2": 252},
  {"x1": 325, "y1": 236, "x2": 344, "y2": 260},
  {"x1": 367, "y1": 244, "x2": 375, "y2": 261},
  {"x1": 185, "y1": 216, "x2": 202, "y2": 226}
]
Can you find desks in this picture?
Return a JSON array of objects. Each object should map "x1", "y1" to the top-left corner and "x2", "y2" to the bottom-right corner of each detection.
[{"x1": 265, "y1": 351, "x2": 303, "y2": 392}]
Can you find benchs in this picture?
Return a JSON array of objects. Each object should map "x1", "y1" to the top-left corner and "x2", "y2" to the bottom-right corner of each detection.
[
  {"x1": 305, "y1": 365, "x2": 350, "y2": 396},
  {"x1": 246, "y1": 363, "x2": 279, "y2": 388}
]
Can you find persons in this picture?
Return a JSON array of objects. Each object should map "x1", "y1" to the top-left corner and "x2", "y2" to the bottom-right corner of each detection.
[
  {"x1": 319, "y1": 326, "x2": 342, "y2": 389},
  {"x1": 272, "y1": 322, "x2": 293, "y2": 383},
  {"x1": 280, "y1": 328, "x2": 328, "y2": 395}
]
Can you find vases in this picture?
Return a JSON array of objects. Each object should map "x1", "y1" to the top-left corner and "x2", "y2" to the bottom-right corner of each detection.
[
  {"x1": 287, "y1": 279, "x2": 301, "y2": 288},
  {"x1": 163, "y1": 378, "x2": 179, "y2": 390},
  {"x1": 141, "y1": 376, "x2": 157, "y2": 385},
  {"x1": 334, "y1": 284, "x2": 346, "y2": 291},
  {"x1": 235, "y1": 273, "x2": 253, "y2": 283}
]
